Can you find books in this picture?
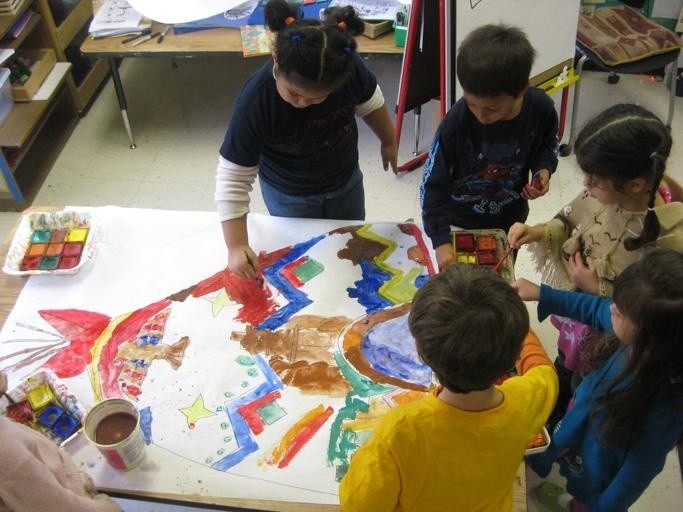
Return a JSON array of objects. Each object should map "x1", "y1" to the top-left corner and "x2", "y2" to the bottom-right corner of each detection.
[
  {"x1": 240, "y1": 25, "x2": 277, "y2": 58},
  {"x1": 0, "y1": 0, "x2": 33, "y2": 41},
  {"x1": 88, "y1": 2, "x2": 152, "y2": 41}
]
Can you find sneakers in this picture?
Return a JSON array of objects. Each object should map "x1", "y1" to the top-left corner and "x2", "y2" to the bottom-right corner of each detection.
[{"x1": 537, "y1": 481, "x2": 573, "y2": 512}]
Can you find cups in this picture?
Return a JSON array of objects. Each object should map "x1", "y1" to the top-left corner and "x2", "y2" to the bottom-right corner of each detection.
[{"x1": 83, "y1": 398, "x2": 146, "y2": 473}]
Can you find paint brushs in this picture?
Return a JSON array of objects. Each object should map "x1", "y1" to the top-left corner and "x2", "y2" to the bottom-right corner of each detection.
[{"x1": 494, "y1": 248, "x2": 513, "y2": 271}]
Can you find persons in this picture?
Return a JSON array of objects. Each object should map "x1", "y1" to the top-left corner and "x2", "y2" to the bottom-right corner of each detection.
[
  {"x1": 420, "y1": 25, "x2": 559, "y2": 269},
  {"x1": 511, "y1": 248, "x2": 683, "y2": 512},
  {"x1": 0, "y1": 373, "x2": 122, "y2": 512},
  {"x1": 215, "y1": 2, "x2": 396, "y2": 280},
  {"x1": 337, "y1": 263, "x2": 560, "y2": 512},
  {"x1": 507, "y1": 104, "x2": 682, "y2": 438}
]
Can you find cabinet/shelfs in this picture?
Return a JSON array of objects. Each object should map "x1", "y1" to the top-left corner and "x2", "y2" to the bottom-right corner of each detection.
[{"x1": 0, "y1": 0, "x2": 118, "y2": 202}]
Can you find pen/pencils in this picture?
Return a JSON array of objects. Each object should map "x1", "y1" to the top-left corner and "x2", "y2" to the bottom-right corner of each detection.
[
  {"x1": 397, "y1": 4, "x2": 409, "y2": 27},
  {"x1": 91, "y1": 25, "x2": 170, "y2": 46}
]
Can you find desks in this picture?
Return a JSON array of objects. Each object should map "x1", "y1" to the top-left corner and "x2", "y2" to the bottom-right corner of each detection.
[
  {"x1": 80, "y1": 24, "x2": 422, "y2": 156},
  {"x1": 0, "y1": 206, "x2": 529, "y2": 512}
]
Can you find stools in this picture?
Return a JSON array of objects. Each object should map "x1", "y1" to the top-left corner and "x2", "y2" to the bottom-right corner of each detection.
[{"x1": 558, "y1": 0, "x2": 680, "y2": 157}]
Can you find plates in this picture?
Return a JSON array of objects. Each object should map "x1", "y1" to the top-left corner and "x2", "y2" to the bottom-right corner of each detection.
[
  {"x1": 2, "y1": 212, "x2": 98, "y2": 275},
  {"x1": 452, "y1": 228, "x2": 516, "y2": 288},
  {"x1": 3, "y1": 368, "x2": 86, "y2": 447}
]
